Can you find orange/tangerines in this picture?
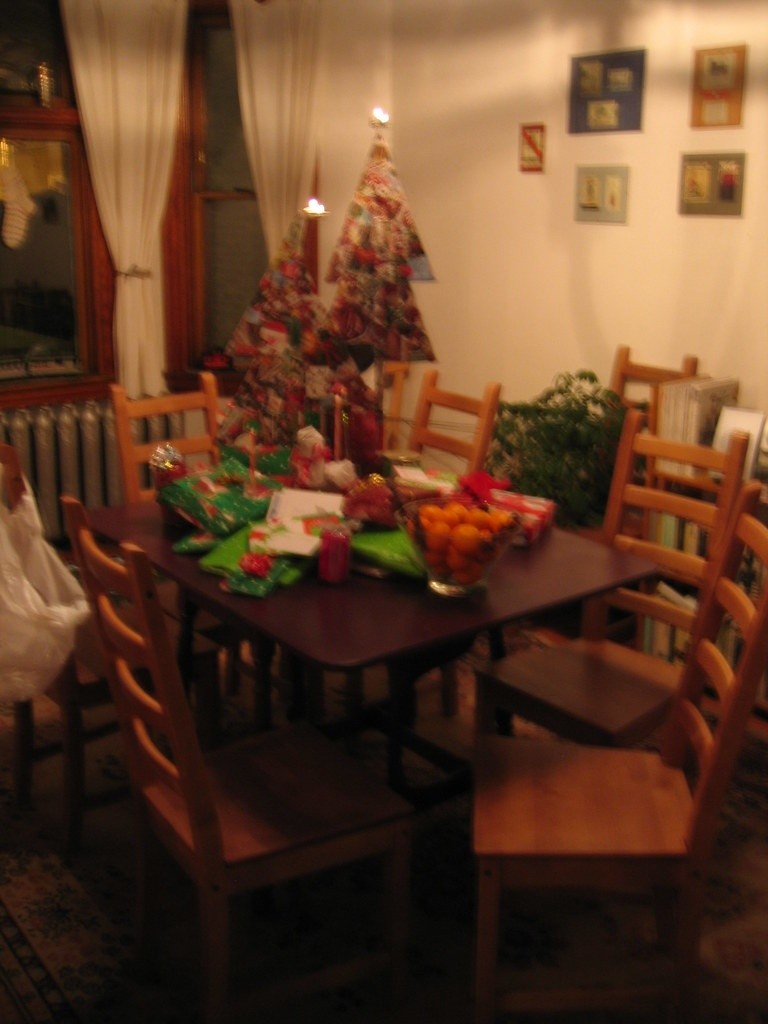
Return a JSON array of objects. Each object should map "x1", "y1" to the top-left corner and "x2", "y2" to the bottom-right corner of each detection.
[{"x1": 412, "y1": 503, "x2": 513, "y2": 584}]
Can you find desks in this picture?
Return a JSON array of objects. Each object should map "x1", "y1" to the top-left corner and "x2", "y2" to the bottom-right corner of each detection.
[{"x1": 87, "y1": 479, "x2": 660, "y2": 932}]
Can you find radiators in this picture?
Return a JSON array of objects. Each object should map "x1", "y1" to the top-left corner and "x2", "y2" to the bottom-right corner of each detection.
[{"x1": 1, "y1": 392, "x2": 182, "y2": 543}]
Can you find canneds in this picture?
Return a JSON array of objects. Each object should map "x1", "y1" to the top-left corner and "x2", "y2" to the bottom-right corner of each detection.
[{"x1": 319, "y1": 526, "x2": 348, "y2": 584}]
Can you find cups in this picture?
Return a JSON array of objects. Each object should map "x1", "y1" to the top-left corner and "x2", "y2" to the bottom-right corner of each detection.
[{"x1": 394, "y1": 496, "x2": 521, "y2": 599}]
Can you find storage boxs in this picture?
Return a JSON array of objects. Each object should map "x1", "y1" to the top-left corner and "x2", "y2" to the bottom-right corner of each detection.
[{"x1": 490, "y1": 489, "x2": 556, "y2": 549}]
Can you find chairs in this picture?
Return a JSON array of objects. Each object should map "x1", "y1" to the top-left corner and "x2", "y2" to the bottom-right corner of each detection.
[{"x1": 2, "y1": 345, "x2": 768, "y2": 1024}]
